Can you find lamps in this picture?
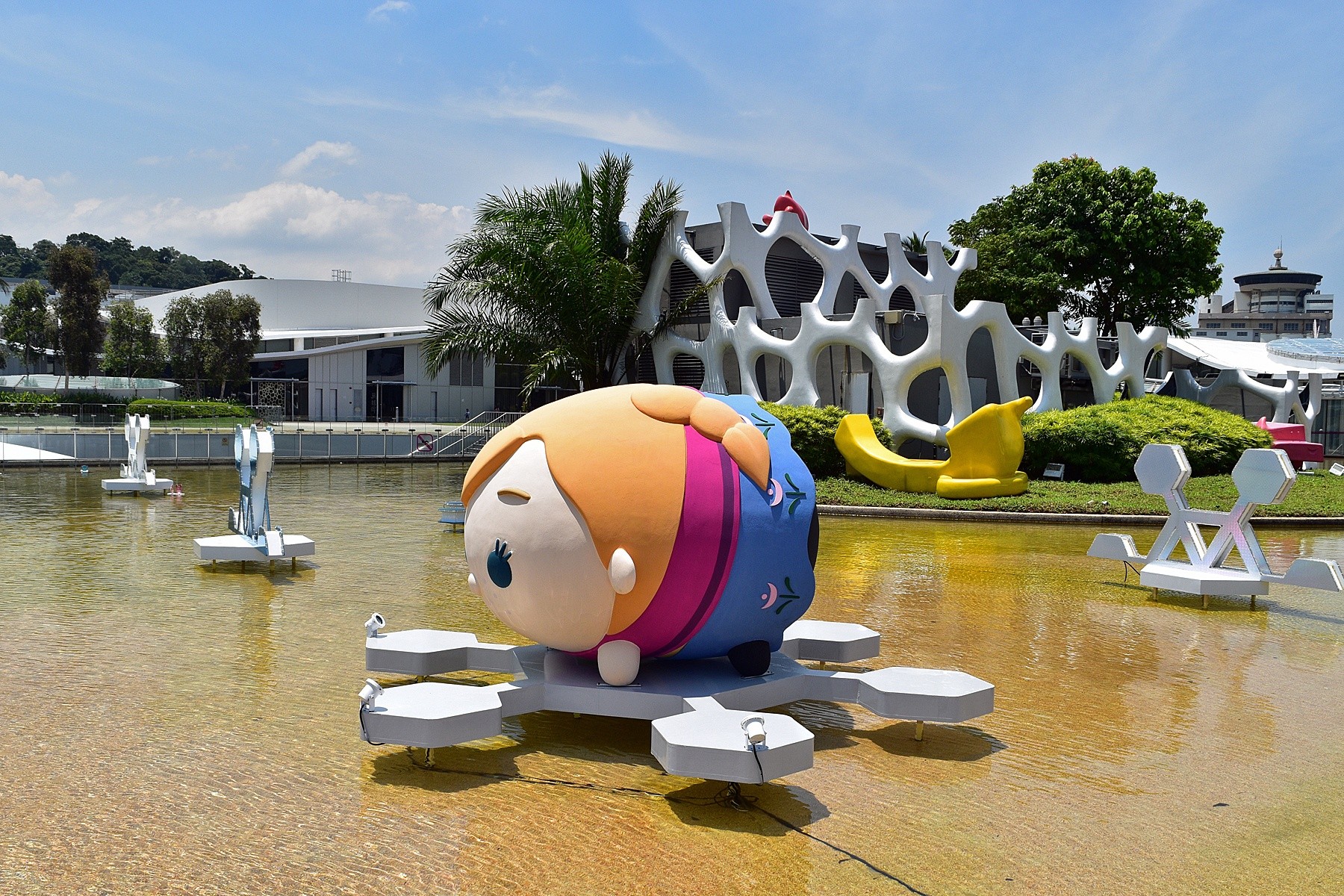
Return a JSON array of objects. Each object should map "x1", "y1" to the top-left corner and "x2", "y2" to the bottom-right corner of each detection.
[
  {"x1": 364, "y1": 612, "x2": 385, "y2": 637},
  {"x1": 1302, "y1": 461, "x2": 1317, "y2": 470},
  {"x1": 358, "y1": 678, "x2": 384, "y2": 712},
  {"x1": 1043, "y1": 463, "x2": 1065, "y2": 481},
  {"x1": 742, "y1": 715, "x2": 767, "y2": 750},
  {"x1": 1329, "y1": 462, "x2": 1344, "y2": 476}
]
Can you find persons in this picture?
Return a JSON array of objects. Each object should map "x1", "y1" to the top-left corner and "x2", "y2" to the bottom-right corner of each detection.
[
  {"x1": 464, "y1": 409, "x2": 470, "y2": 421},
  {"x1": 520, "y1": 401, "x2": 535, "y2": 417}
]
